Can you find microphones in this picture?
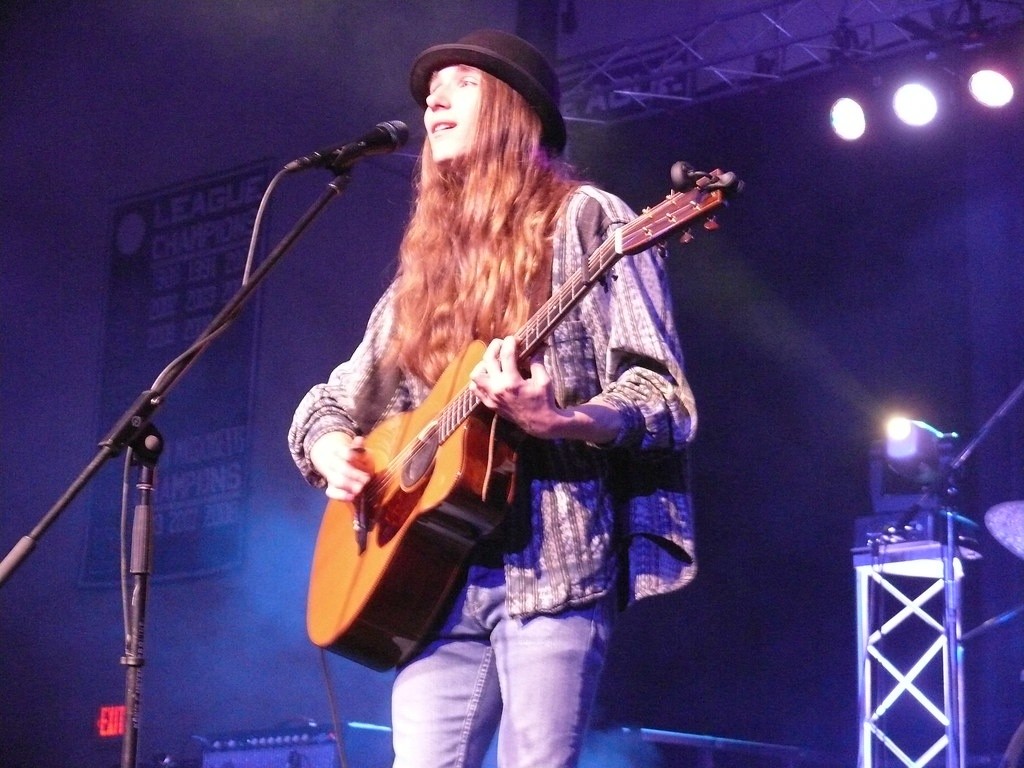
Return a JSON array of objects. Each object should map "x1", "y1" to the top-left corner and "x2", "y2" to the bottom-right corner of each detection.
[{"x1": 284, "y1": 120, "x2": 410, "y2": 173}]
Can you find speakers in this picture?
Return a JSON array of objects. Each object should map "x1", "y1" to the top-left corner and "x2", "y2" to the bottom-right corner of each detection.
[{"x1": 201, "y1": 721, "x2": 393, "y2": 768}]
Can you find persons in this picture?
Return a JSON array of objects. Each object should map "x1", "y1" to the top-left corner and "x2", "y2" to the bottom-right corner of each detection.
[
  {"x1": 984, "y1": 450, "x2": 1024, "y2": 561},
  {"x1": 287, "y1": 28, "x2": 697, "y2": 768}
]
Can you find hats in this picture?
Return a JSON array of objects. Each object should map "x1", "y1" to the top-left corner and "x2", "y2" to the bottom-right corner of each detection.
[{"x1": 409, "y1": 28, "x2": 566, "y2": 160}]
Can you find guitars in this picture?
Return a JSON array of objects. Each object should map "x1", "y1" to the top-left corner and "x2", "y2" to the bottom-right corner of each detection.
[{"x1": 299, "y1": 155, "x2": 744, "y2": 677}]
